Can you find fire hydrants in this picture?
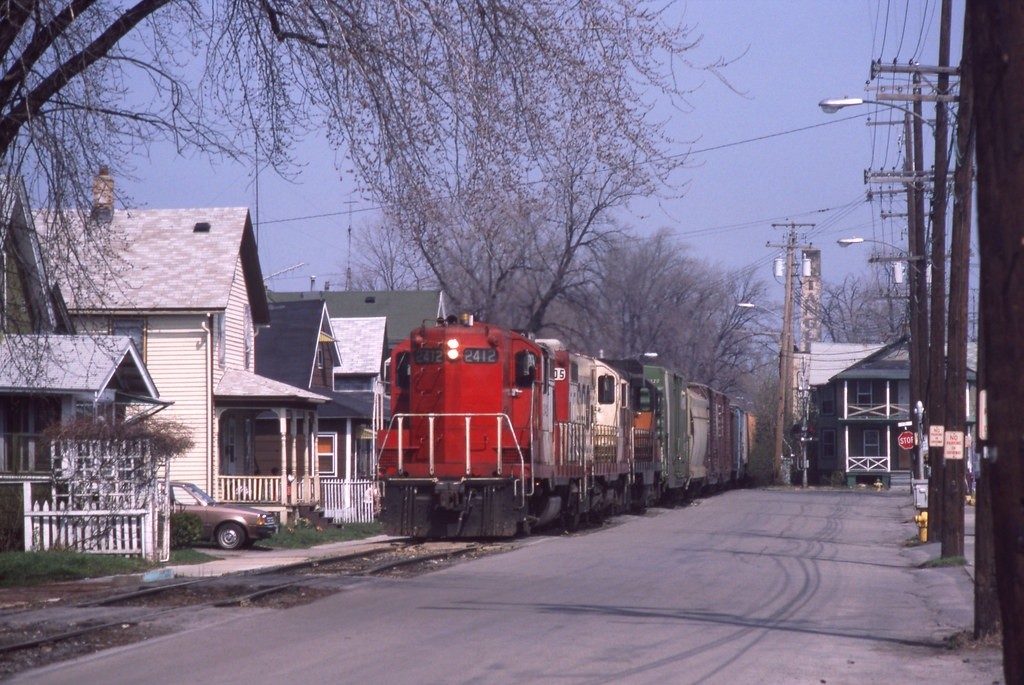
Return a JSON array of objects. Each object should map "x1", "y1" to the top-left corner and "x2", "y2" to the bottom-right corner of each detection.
[{"x1": 914, "y1": 513, "x2": 930, "y2": 546}]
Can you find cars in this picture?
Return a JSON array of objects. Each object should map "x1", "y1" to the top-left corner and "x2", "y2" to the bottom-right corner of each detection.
[{"x1": 152, "y1": 481, "x2": 277, "y2": 552}]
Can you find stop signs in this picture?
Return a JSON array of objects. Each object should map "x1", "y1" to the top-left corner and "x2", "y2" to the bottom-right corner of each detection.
[{"x1": 898, "y1": 430, "x2": 915, "y2": 451}]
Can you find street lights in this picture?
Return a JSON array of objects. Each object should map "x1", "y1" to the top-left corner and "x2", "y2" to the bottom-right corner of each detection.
[
  {"x1": 836, "y1": 237, "x2": 924, "y2": 493},
  {"x1": 738, "y1": 301, "x2": 795, "y2": 461},
  {"x1": 820, "y1": 97, "x2": 944, "y2": 541}
]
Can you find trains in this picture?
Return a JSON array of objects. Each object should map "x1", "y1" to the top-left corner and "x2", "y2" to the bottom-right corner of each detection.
[{"x1": 371, "y1": 312, "x2": 763, "y2": 552}]
